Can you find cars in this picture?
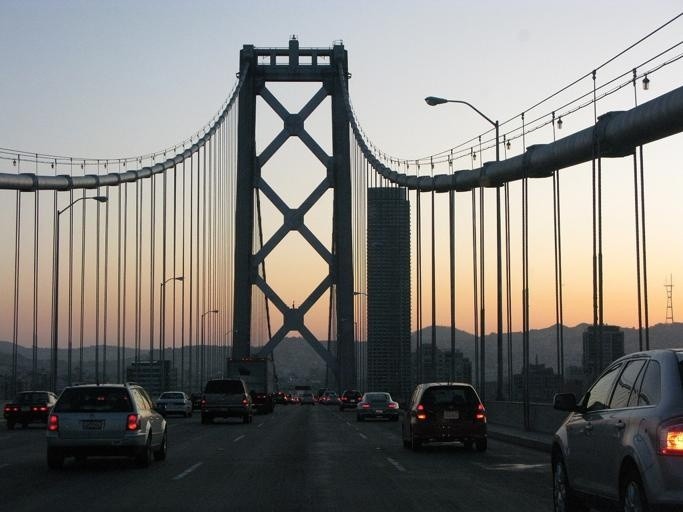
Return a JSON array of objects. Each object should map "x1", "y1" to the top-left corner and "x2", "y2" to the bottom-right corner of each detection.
[
  {"x1": 3, "y1": 382, "x2": 168, "y2": 467},
  {"x1": 552, "y1": 349, "x2": 683, "y2": 512},
  {"x1": 403, "y1": 383, "x2": 487, "y2": 452},
  {"x1": 157, "y1": 379, "x2": 399, "y2": 424}
]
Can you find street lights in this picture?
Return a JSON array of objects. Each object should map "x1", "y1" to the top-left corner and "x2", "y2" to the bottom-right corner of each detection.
[
  {"x1": 425, "y1": 96, "x2": 503, "y2": 399},
  {"x1": 54, "y1": 197, "x2": 107, "y2": 390},
  {"x1": 201, "y1": 311, "x2": 218, "y2": 393},
  {"x1": 160, "y1": 277, "x2": 183, "y2": 392}
]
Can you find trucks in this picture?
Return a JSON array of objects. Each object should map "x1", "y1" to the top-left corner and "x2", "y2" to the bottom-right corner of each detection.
[{"x1": 225, "y1": 357, "x2": 275, "y2": 413}]
